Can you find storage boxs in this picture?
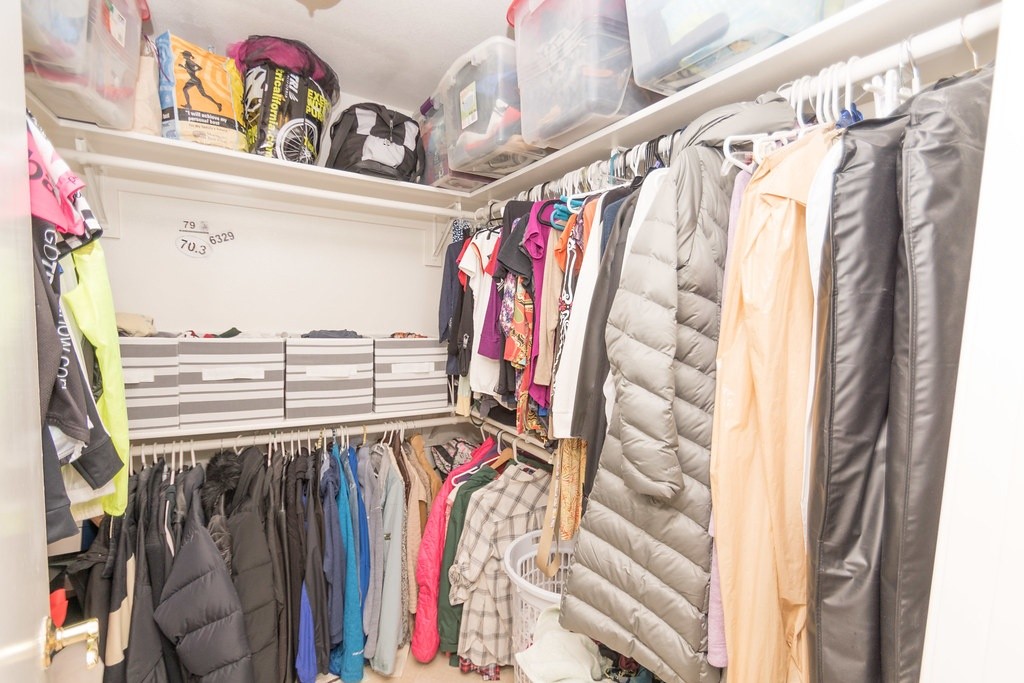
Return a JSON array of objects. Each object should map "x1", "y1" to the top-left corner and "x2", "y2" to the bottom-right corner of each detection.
[
  {"x1": 505, "y1": 0, "x2": 668, "y2": 151},
  {"x1": 117, "y1": 333, "x2": 448, "y2": 433},
  {"x1": 22, "y1": 0, "x2": 152, "y2": 131},
  {"x1": 623, "y1": 0, "x2": 862, "y2": 95},
  {"x1": 411, "y1": 35, "x2": 550, "y2": 193}
]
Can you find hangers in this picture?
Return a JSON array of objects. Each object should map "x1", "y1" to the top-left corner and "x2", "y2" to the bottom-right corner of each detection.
[
  {"x1": 473, "y1": 126, "x2": 688, "y2": 240},
  {"x1": 128, "y1": 416, "x2": 417, "y2": 485},
  {"x1": 722, "y1": 14, "x2": 977, "y2": 177},
  {"x1": 450, "y1": 421, "x2": 537, "y2": 486}
]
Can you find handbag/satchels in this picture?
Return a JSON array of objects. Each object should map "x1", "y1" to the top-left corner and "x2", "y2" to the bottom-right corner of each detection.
[
  {"x1": 244, "y1": 63, "x2": 331, "y2": 164},
  {"x1": 325, "y1": 102, "x2": 425, "y2": 182}
]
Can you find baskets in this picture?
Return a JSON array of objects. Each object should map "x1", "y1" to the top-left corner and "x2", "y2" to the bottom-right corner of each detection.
[{"x1": 503, "y1": 528, "x2": 601, "y2": 683}]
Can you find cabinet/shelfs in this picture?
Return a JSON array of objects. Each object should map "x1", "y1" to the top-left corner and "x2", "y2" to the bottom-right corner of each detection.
[{"x1": 22, "y1": 0, "x2": 1006, "y2": 683}]
